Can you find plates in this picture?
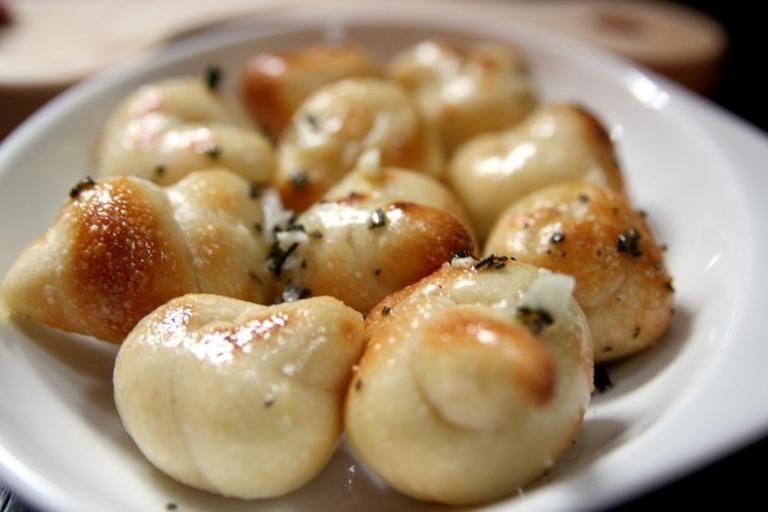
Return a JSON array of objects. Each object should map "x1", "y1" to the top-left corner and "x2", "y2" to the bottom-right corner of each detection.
[{"x1": 2, "y1": 0, "x2": 766, "y2": 512}]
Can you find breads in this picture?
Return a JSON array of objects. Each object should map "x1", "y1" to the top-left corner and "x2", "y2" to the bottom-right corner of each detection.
[{"x1": 0, "y1": 38, "x2": 677, "y2": 506}]
